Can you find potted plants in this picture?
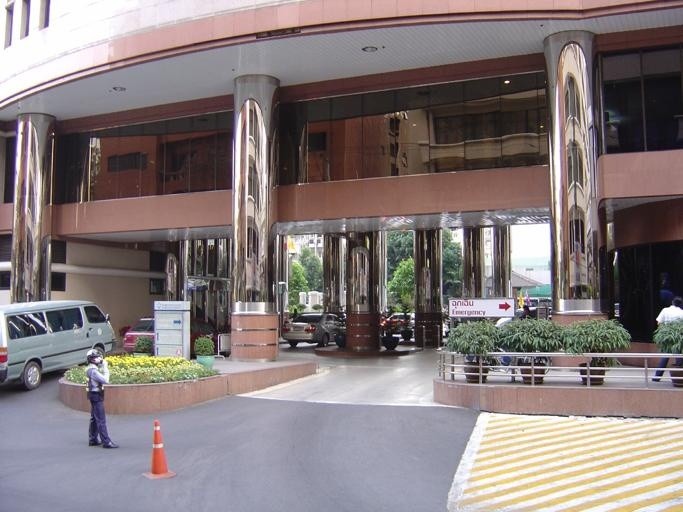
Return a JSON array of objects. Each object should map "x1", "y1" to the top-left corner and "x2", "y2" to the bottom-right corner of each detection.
[
  {"x1": 381, "y1": 303, "x2": 412, "y2": 350},
  {"x1": 131, "y1": 335, "x2": 152, "y2": 358},
  {"x1": 333, "y1": 303, "x2": 346, "y2": 347},
  {"x1": 194, "y1": 335, "x2": 216, "y2": 369},
  {"x1": 451, "y1": 317, "x2": 682, "y2": 388}
]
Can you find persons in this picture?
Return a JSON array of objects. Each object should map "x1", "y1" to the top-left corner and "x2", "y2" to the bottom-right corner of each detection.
[
  {"x1": 86, "y1": 349, "x2": 119, "y2": 448},
  {"x1": 652, "y1": 296, "x2": 683, "y2": 381},
  {"x1": 519, "y1": 305, "x2": 535, "y2": 321}
]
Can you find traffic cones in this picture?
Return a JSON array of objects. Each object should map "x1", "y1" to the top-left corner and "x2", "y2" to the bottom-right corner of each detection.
[{"x1": 142, "y1": 419, "x2": 176, "y2": 480}]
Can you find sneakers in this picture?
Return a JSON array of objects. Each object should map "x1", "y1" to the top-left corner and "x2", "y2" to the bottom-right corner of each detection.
[
  {"x1": 89, "y1": 441, "x2": 102, "y2": 446},
  {"x1": 103, "y1": 441, "x2": 118, "y2": 448}
]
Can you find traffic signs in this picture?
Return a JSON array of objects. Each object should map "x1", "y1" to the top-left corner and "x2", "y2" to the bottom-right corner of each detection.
[{"x1": 449, "y1": 298, "x2": 514, "y2": 319}]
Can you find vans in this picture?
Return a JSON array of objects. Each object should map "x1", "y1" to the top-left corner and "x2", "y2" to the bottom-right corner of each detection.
[{"x1": 0, "y1": 299, "x2": 116, "y2": 391}]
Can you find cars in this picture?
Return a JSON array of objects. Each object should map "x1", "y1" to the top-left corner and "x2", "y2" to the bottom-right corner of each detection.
[
  {"x1": 333, "y1": 312, "x2": 387, "y2": 347},
  {"x1": 122, "y1": 315, "x2": 219, "y2": 359},
  {"x1": 494, "y1": 305, "x2": 553, "y2": 333},
  {"x1": 282, "y1": 311, "x2": 347, "y2": 347},
  {"x1": 381, "y1": 310, "x2": 417, "y2": 339}
]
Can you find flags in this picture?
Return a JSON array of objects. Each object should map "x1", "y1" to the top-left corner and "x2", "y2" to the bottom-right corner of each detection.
[
  {"x1": 524, "y1": 289, "x2": 531, "y2": 306},
  {"x1": 518, "y1": 289, "x2": 524, "y2": 307}
]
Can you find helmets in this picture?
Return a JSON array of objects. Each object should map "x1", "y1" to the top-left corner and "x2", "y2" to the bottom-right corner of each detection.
[{"x1": 86, "y1": 349, "x2": 101, "y2": 358}]
